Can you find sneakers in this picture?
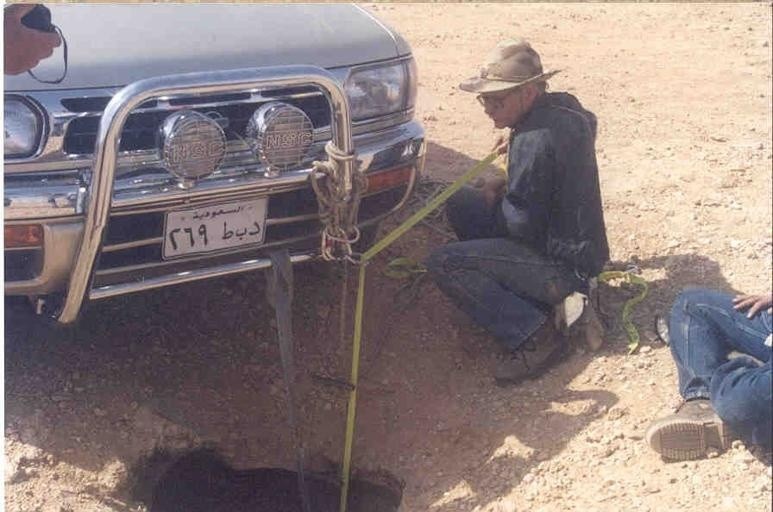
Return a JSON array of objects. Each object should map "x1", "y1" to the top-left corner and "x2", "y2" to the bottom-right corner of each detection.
[
  {"x1": 492, "y1": 321, "x2": 572, "y2": 386},
  {"x1": 643, "y1": 396, "x2": 738, "y2": 462}
]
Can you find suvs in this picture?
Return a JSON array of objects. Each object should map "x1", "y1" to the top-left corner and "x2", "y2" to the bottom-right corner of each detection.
[{"x1": 5, "y1": 3, "x2": 430, "y2": 342}]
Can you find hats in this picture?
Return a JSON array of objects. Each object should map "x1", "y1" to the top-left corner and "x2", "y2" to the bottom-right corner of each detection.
[{"x1": 458, "y1": 36, "x2": 564, "y2": 94}]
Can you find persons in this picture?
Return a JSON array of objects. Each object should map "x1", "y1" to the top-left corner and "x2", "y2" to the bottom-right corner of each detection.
[
  {"x1": 425, "y1": 34, "x2": 612, "y2": 387},
  {"x1": 642, "y1": 287, "x2": 772, "y2": 462},
  {"x1": 3, "y1": 1, "x2": 62, "y2": 77}
]
